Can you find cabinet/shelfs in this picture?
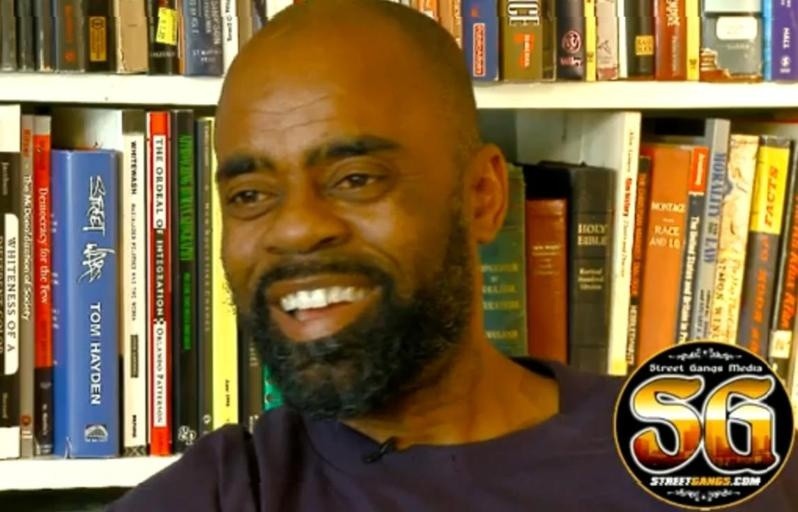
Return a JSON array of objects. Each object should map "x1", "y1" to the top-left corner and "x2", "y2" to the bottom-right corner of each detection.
[{"x1": 0, "y1": 71, "x2": 798, "y2": 492}]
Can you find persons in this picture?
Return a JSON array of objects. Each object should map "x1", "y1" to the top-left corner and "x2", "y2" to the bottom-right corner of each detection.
[{"x1": 103, "y1": 0, "x2": 797, "y2": 511}]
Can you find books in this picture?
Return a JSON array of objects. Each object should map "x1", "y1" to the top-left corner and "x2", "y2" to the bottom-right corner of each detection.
[{"x1": 0, "y1": 0, "x2": 798, "y2": 462}]
